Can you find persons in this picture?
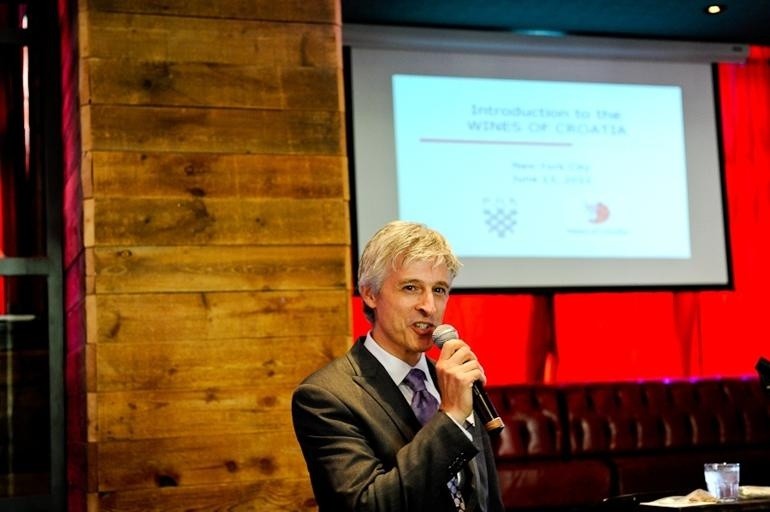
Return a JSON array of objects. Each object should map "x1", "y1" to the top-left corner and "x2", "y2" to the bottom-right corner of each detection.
[{"x1": 290, "y1": 219, "x2": 509, "y2": 512}]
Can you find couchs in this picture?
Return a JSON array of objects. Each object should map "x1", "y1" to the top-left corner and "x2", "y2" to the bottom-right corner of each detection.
[{"x1": 479, "y1": 376, "x2": 770, "y2": 511}]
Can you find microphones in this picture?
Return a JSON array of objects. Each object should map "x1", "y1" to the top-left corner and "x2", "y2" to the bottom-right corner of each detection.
[{"x1": 431, "y1": 324, "x2": 506, "y2": 432}]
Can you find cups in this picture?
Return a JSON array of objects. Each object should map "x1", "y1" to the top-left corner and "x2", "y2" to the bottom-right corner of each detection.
[{"x1": 703, "y1": 463, "x2": 742, "y2": 504}]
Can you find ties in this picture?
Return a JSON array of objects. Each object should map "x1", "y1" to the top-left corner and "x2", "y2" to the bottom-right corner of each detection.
[{"x1": 403, "y1": 369, "x2": 466, "y2": 512}]
